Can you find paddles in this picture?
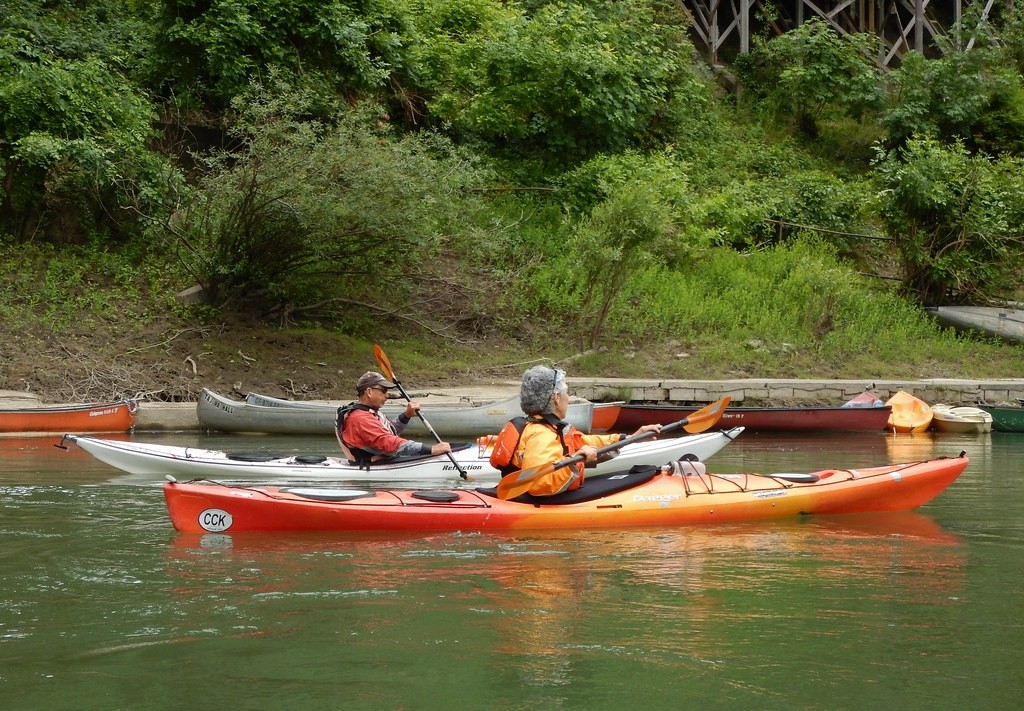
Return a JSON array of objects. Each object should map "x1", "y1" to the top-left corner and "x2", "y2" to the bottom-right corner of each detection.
[{"x1": 373, "y1": 343, "x2": 474, "y2": 483}]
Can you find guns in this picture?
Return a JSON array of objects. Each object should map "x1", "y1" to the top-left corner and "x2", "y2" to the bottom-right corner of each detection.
[{"x1": 497, "y1": 395, "x2": 732, "y2": 500}]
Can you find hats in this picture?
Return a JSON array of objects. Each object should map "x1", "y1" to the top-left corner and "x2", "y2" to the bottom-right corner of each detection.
[{"x1": 356, "y1": 371, "x2": 397, "y2": 391}]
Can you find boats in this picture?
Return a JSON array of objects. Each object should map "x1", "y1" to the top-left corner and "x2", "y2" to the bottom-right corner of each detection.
[
  {"x1": 881, "y1": 391, "x2": 933, "y2": 432},
  {"x1": 977, "y1": 402, "x2": 1024, "y2": 432},
  {"x1": 839, "y1": 391, "x2": 885, "y2": 408},
  {"x1": 927, "y1": 306, "x2": 1024, "y2": 343},
  {"x1": 196, "y1": 388, "x2": 595, "y2": 437},
  {"x1": 66, "y1": 423, "x2": 745, "y2": 479},
  {"x1": 930, "y1": 402, "x2": 994, "y2": 434},
  {"x1": 1, "y1": 398, "x2": 145, "y2": 435},
  {"x1": 161, "y1": 452, "x2": 970, "y2": 534},
  {"x1": 611, "y1": 404, "x2": 891, "y2": 433},
  {"x1": 591, "y1": 399, "x2": 625, "y2": 431}
]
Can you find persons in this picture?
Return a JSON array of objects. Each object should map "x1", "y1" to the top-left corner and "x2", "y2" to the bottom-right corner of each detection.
[
  {"x1": 501, "y1": 366, "x2": 663, "y2": 497},
  {"x1": 334, "y1": 371, "x2": 450, "y2": 472}
]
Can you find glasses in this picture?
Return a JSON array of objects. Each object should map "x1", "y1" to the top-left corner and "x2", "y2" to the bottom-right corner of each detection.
[{"x1": 371, "y1": 387, "x2": 388, "y2": 394}]
